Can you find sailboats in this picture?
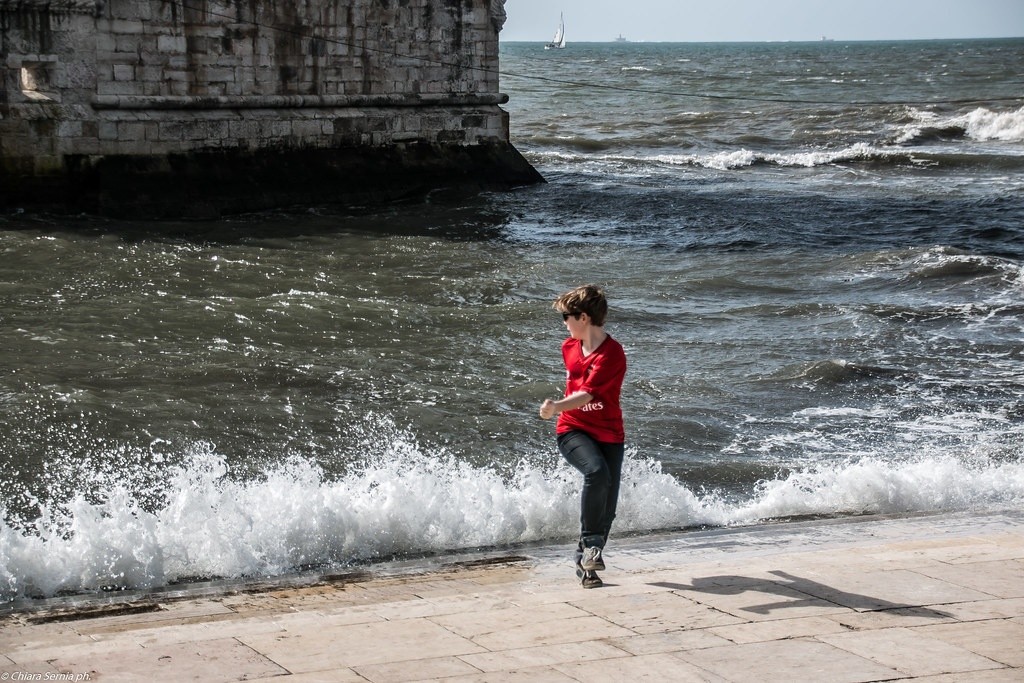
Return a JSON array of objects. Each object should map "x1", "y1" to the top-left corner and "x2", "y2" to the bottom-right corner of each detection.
[
  {"x1": 614, "y1": 34, "x2": 627, "y2": 42},
  {"x1": 544, "y1": 11, "x2": 566, "y2": 50}
]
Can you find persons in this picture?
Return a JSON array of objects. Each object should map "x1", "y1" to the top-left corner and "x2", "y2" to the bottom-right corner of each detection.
[{"x1": 539, "y1": 282, "x2": 628, "y2": 589}]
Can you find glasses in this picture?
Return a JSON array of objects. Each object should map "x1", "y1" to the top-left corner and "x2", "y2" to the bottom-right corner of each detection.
[{"x1": 562, "y1": 311, "x2": 577, "y2": 322}]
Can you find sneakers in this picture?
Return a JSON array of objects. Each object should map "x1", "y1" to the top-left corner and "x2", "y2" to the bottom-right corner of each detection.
[
  {"x1": 576, "y1": 568, "x2": 602, "y2": 589},
  {"x1": 580, "y1": 546, "x2": 605, "y2": 570}
]
